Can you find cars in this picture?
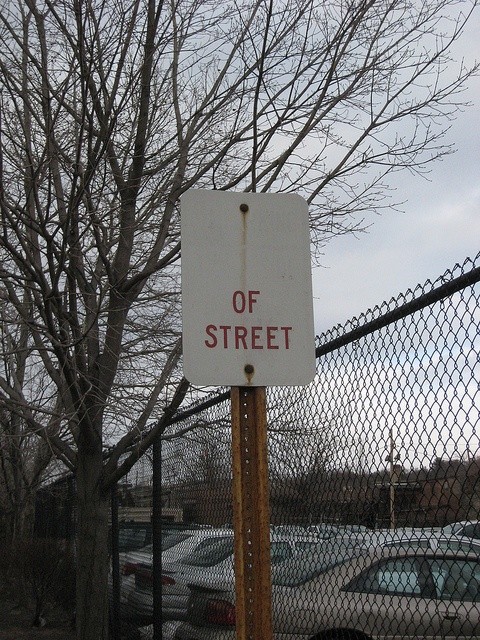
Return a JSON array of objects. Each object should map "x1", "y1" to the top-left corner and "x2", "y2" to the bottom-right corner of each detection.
[
  {"x1": 111, "y1": 530, "x2": 232, "y2": 600},
  {"x1": 176, "y1": 532, "x2": 479, "y2": 640},
  {"x1": 307, "y1": 525, "x2": 339, "y2": 532},
  {"x1": 438, "y1": 521, "x2": 479, "y2": 539},
  {"x1": 128, "y1": 534, "x2": 327, "y2": 619},
  {"x1": 339, "y1": 524, "x2": 369, "y2": 532},
  {"x1": 276, "y1": 526, "x2": 305, "y2": 532}
]
